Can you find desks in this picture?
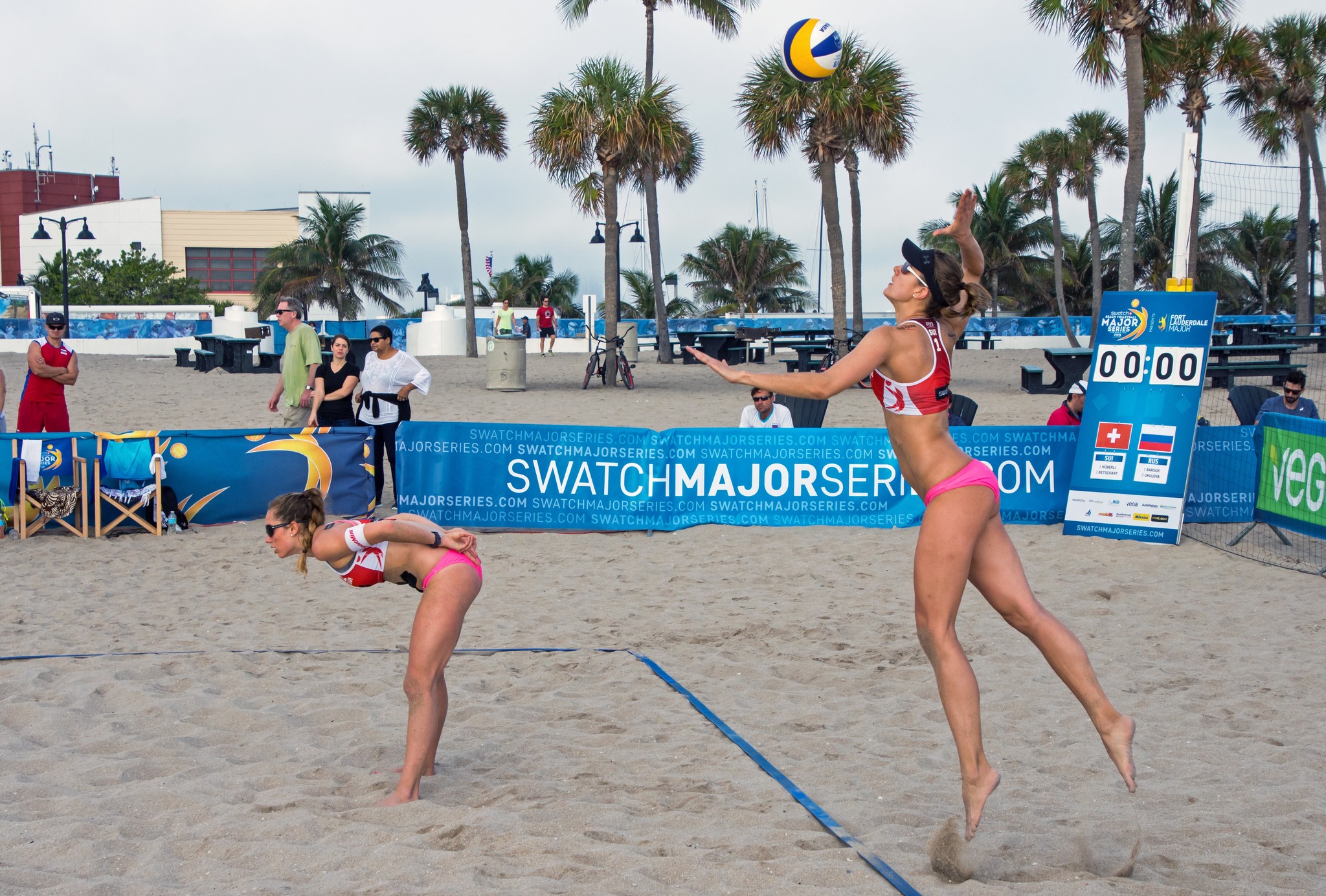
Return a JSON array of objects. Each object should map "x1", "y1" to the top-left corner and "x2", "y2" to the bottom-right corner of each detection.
[
  {"x1": 330, "y1": 338, "x2": 373, "y2": 372},
  {"x1": 1043, "y1": 347, "x2": 1094, "y2": 395},
  {"x1": 955, "y1": 330, "x2": 1000, "y2": 350},
  {"x1": 670, "y1": 331, "x2": 739, "y2": 359},
  {"x1": 214, "y1": 337, "x2": 262, "y2": 374},
  {"x1": 847, "y1": 330, "x2": 870, "y2": 333},
  {"x1": 1270, "y1": 323, "x2": 1326, "y2": 354},
  {"x1": 1205, "y1": 344, "x2": 1302, "y2": 393},
  {"x1": 694, "y1": 333, "x2": 746, "y2": 366},
  {"x1": 1226, "y1": 322, "x2": 1266, "y2": 357},
  {"x1": 637, "y1": 333, "x2": 677, "y2": 350},
  {"x1": 193, "y1": 334, "x2": 234, "y2": 368},
  {"x1": 786, "y1": 343, "x2": 854, "y2": 373},
  {"x1": 762, "y1": 328, "x2": 834, "y2": 356}
]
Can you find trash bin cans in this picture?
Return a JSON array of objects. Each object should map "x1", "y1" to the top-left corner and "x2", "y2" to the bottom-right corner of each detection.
[
  {"x1": 485, "y1": 333, "x2": 528, "y2": 392},
  {"x1": 617, "y1": 322, "x2": 640, "y2": 363}
]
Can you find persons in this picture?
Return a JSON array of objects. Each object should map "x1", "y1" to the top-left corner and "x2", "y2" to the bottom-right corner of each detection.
[
  {"x1": 0, "y1": 320, "x2": 19, "y2": 339},
  {"x1": 537, "y1": 326, "x2": 539, "y2": 328},
  {"x1": 1047, "y1": 380, "x2": 1089, "y2": 426},
  {"x1": 944, "y1": 387, "x2": 966, "y2": 426},
  {"x1": 686, "y1": 189, "x2": 1139, "y2": 843},
  {"x1": 267, "y1": 296, "x2": 322, "y2": 428},
  {"x1": 307, "y1": 334, "x2": 360, "y2": 428},
  {"x1": 739, "y1": 386, "x2": 794, "y2": 428},
  {"x1": 536, "y1": 296, "x2": 558, "y2": 358},
  {"x1": 67, "y1": 321, "x2": 121, "y2": 340},
  {"x1": 308, "y1": 321, "x2": 317, "y2": 334},
  {"x1": 1254, "y1": 369, "x2": 1321, "y2": 426},
  {"x1": 265, "y1": 488, "x2": 482, "y2": 806},
  {"x1": 22, "y1": 320, "x2": 46, "y2": 339},
  {"x1": 519, "y1": 316, "x2": 531, "y2": 339},
  {"x1": 0, "y1": 312, "x2": 79, "y2": 433},
  {"x1": 495, "y1": 299, "x2": 517, "y2": 335},
  {"x1": 123, "y1": 320, "x2": 197, "y2": 339},
  {"x1": 355, "y1": 325, "x2": 431, "y2": 511}
]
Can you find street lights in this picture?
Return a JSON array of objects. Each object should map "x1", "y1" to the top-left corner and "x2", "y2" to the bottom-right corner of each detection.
[
  {"x1": 31, "y1": 216, "x2": 97, "y2": 339},
  {"x1": 1284, "y1": 218, "x2": 1320, "y2": 333},
  {"x1": 587, "y1": 221, "x2": 647, "y2": 323}
]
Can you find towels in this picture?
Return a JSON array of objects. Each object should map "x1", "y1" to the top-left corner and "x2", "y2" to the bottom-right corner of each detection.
[
  {"x1": 149, "y1": 453, "x2": 166, "y2": 480},
  {"x1": 20, "y1": 439, "x2": 42, "y2": 482},
  {"x1": 8, "y1": 457, "x2": 28, "y2": 506}
]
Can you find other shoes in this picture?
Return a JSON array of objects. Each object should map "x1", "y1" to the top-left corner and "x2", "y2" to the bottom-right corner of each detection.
[
  {"x1": 541, "y1": 353, "x2": 546, "y2": 357},
  {"x1": 548, "y1": 349, "x2": 554, "y2": 356},
  {"x1": 390, "y1": 500, "x2": 398, "y2": 510},
  {"x1": 374, "y1": 501, "x2": 383, "y2": 508}
]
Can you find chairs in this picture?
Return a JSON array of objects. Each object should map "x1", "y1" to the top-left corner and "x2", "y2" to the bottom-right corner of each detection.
[
  {"x1": 948, "y1": 394, "x2": 978, "y2": 426},
  {"x1": 1227, "y1": 385, "x2": 1296, "y2": 548},
  {"x1": 94, "y1": 436, "x2": 162, "y2": 538},
  {"x1": 8, "y1": 436, "x2": 89, "y2": 539},
  {"x1": 774, "y1": 393, "x2": 829, "y2": 428}
]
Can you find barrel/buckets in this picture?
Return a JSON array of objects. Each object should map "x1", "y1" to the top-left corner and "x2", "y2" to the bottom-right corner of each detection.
[
  {"x1": 486, "y1": 333, "x2": 527, "y2": 392},
  {"x1": 713, "y1": 324, "x2": 737, "y2": 338},
  {"x1": 616, "y1": 322, "x2": 638, "y2": 363}
]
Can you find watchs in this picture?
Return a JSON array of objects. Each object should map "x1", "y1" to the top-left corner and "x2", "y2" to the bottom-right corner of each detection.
[
  {"x1": 555, "y1": 325, "x2": 558, "y2": 327},
  {"x1": 428, "y1": 527, "x2": 441, "y2": 550},
  {"x1": 305, "y1": 385, "x2": 314, "y2": 391}
]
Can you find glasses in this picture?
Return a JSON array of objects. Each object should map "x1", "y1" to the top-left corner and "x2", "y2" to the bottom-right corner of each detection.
[
  {"x1": 132, "y1": 331, "x2": 138, "y2": 333},
  {"x1": 6, "y1": 325, "x2": 12, "y2": 328},
  {"x1": 752, "y1": 395, "x2": 771, "y2": 402},
  {"x1": 79, "y1": 325, "x2": 85, "y2": 328},
  {"x1": 368, "y1": 337, "x2": 386, "y2": 343},
  {"x1": 901, "y1": 261, "x2": 929, "y2": 289},
  {"x1": 544, "y1": 301, "x2": 549, "y2": 303},
  {"x1": 47, "y1": 324, "x2": 65, "y2": 330},
  {"x1": 504, "y1": 302, "x2": 509, "y2": 304},
  {"x1": 107, "y1": 326, "x2": 113, "y2": 328},
  {"x1": 184, "y1": 327, "x2": 189, "y2": 329},
  {"x1": 265, "y1": 521, "x2": 302, "y2": 537},
  {"x1": 1283, "y1": 385, "x2": 1303, "y2": 395},
  {"x1": 275, "y1": 309, "x2": 293, "y2": 315},
  {"x1": 154, "y1": 325, "x2": 161, "y2": 328}
]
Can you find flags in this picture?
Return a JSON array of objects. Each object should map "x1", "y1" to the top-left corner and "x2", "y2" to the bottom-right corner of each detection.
[{"x1": 485, "y1": 255, "x2": 492, "y2": 277}]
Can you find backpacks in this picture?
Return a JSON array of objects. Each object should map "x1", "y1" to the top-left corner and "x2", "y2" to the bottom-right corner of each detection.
[{"x1": 145, "y1": 486, "x2": 188, "y2": 532}]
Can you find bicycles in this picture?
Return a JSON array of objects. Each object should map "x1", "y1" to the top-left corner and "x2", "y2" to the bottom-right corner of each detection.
[
  {"x1": 818, "y1": 327, "x2": 859, "y2": 373},
  {"x1": 582, "y1": 325, "x2": 620, "y2": 389},
  {"x1": 415, "y1": 272, "x2": 436, "y2": 312},
  {"x1": 842, "y1": 327, "x2": 873, "y2": 389},
  {"x1": 600, "y1": 325, "x2": 636, "y2": 390}
]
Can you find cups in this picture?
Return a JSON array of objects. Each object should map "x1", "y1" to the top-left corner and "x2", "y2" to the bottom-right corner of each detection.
[{"x1": 519, "y1": 327, "x2": 522, "y2": 332}]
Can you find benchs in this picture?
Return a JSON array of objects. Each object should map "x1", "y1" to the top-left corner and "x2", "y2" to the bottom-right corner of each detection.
[
  {"x1": 320, "y1": 351, "x2": 334, "y2": 363},
  {"x1": 637, "y1": 337, "x2": 858, "y2": 370},
  {"x1": 193, "y1": 349, "x2": 216, "y2": 374},
  {"x1": 1206, "y1": 332, "x2": 1326, "y2": 378},
  {"x1": 257, "y1": 351, "x2": 283, "y2": 374},
  {"x1": 174, "y1": 347, "x2": 191, "y2": 368},
  {"x1": 1020, "y1": 365, "x2": 1044, "y2": 395},
  {"x1": 957, "y1": 339, "x2": 1002, "y2": 342}
]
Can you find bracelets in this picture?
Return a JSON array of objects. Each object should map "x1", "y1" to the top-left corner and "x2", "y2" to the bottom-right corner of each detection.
[{"x1": 514, "y1": 326, "x2": 517, "y2": 327}]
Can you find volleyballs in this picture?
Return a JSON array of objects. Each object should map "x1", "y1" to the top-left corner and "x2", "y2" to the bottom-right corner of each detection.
[{"x1": 780, "y1": 18, "x2": 842, "y2": 83}]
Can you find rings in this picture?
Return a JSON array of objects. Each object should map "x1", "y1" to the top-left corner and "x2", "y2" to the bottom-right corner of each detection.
[
  {"x1": 312, "y1": 392, "x2": 313, "y2": 395},
  {"x1": 402, "y1": 399, "x2": 405, "y2": 401}
]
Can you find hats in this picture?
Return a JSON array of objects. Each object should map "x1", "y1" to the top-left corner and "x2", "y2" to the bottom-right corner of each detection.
[
  {"x1": 6, "y1": 321, "x2": 16, "y2": 329},
  {"x1": 901, "y1": 238, "x2": 949, "y2": 309},
  {"x1": 32, "y1": 320, "x2": 43, "y2": 326},
  {"x1": 108, "y1": 322, "x2": 115, "y2": 327},
  {"x1": 521, "y1": 316, "x2": 529, "y2": 320},
  {"x1": 186, "y1": 325, "x2": 193, "y2": 330},
  {"x1": 1069, "y1": 380, "x2": 1091, "y2": 396},
  {"x1": 46, "y1": 312, "x2": 66, "y2": 324},
  {"x1": 153, "y1": 320, "x2": 161, "y2": 326}
]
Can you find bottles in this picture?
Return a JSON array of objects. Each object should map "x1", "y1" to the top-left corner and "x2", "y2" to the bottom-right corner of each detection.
[
  {"x1": 9, "y1": 529, "x2": 19, "y2": 540},
  {"x1": 167, "y1": 511, "x2": 177, "y2": 536},
  {"x1": 0, "y1": 514, "x2": 5, "y2": 539}
]
